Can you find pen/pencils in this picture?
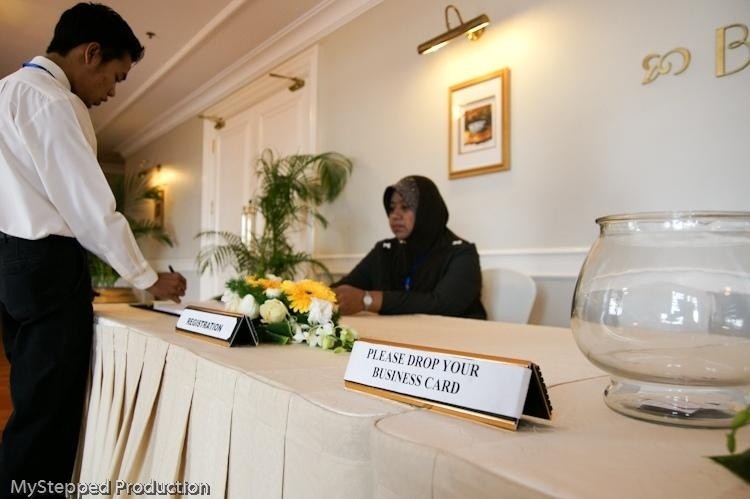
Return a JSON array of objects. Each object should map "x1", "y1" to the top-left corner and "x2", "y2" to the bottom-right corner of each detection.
[{"x1": 169, "y1": 265, "x2": 174, "y2": 272}]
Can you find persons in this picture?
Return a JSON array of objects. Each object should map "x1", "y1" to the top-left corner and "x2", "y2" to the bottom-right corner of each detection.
[
  {"x1": 1, "y1": 0, "x2": 188, "y2": 499},
  {"x1": 327, "y1": 176, "x2": 490, "y2": 322}
]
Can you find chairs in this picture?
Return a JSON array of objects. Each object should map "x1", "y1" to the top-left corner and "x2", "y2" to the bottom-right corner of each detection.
[{"x1": 479, "y1": 266, "x2": 538, "y2": 324}]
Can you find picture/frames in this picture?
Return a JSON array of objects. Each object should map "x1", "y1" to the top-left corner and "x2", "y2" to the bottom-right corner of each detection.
[{"x1": 447, "y1": 66, "x2": 511, "y2": 182}]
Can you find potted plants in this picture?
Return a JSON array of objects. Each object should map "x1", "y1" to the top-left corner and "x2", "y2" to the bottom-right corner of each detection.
[{"x1": 131, "y1": 187, "x2": 160, "y2": 222}]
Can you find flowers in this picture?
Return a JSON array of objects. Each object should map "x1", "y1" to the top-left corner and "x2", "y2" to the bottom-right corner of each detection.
[{"x1": 222, "y1": 275, "x2": 358, "y2": 354}]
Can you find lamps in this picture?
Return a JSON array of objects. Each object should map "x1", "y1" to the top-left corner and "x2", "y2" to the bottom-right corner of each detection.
[
  {"x1": 137, "y1": 160, "x2": 162, "y2": 178},
  {"x1": 415, "y1": 5, "x2": 491, "y2": 56}
]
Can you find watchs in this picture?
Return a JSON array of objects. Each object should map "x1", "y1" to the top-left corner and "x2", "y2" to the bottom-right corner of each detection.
[{"x1": 362, "y1": 288, "x2": 374, "y2": 310}]
